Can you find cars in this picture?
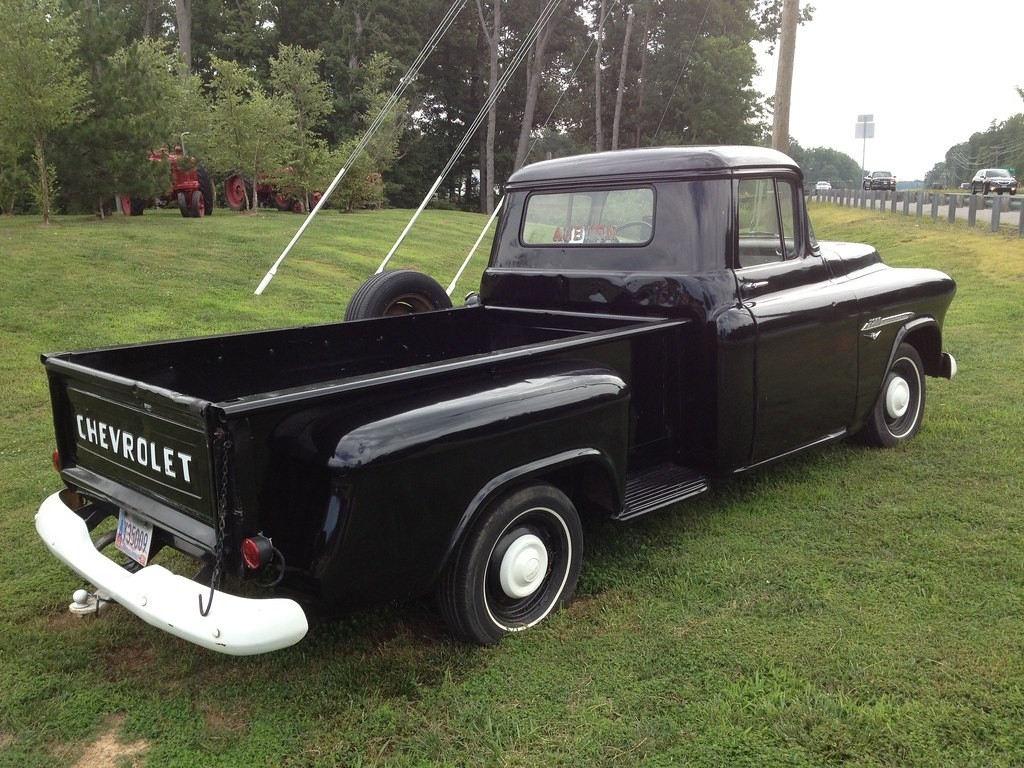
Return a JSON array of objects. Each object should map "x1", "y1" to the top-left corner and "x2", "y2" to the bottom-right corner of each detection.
[
  {"x1": 958, "y1": 183, "x2": 970, "y2": 190},
  {"x1": 931, "y1": 181, "x2": 947, "y2": 190},
  {"x1": 815, "y1": 181, "x2": 832, "y2": 190},
  {"x1": 970, "y1": 169, "x2": 1018, "y2": 196}
]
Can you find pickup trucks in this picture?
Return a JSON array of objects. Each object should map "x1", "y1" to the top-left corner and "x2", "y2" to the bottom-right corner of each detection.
[
  {"x1": 33, "y1": 145, "x2": 956, "y2": 657},
  {"x1": 863, "y1": 170, "x2": 896, "y2": 190}
]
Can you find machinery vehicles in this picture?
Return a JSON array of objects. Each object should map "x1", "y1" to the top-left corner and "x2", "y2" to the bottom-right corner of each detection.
[
  {"x1": 120, "y1": 131, "x2": 216, "y2": 217},
  {"x1": 226, "y1": 157, "x2": 326, "y2": 213}
]
